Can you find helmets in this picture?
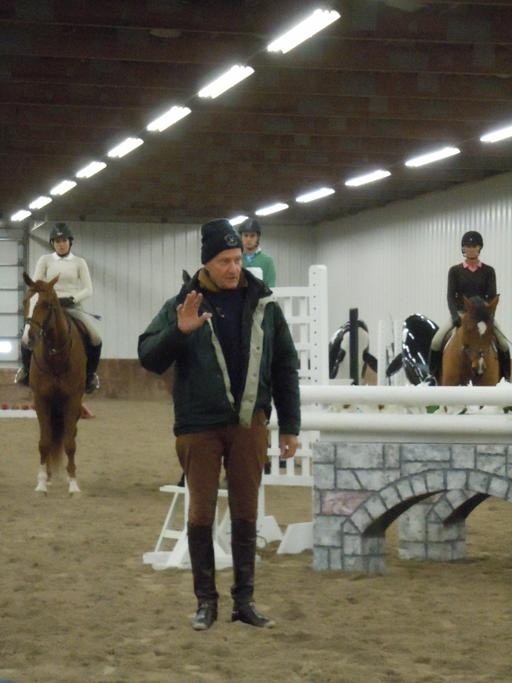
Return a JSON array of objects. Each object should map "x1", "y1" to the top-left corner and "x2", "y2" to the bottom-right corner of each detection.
[
  {"x1": 460, "y1": 230, "x2": 485, "y2": 249},
  {"x1": 238, "y1": 217, "x2": 264, "y2": 237},
  {"x1": 48, "y1": 222, "x2": 76, "y2": 241}
]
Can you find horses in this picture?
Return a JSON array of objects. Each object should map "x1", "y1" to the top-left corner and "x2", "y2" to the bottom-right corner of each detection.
[
  {"x1": 15, "y1": 269, "x2": 92, "y2": 501},
  {"x1": 436, "y1": 291, "x2": 502, "y2": 386}
]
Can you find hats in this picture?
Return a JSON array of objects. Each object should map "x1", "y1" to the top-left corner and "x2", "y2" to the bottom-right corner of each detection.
[{"x1": 198, "y1": 218, "x2": 244, "y2": 263}]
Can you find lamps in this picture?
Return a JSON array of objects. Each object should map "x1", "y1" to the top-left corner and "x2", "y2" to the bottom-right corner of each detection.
[
  {"x1": 228, "y1": 214, "x2": 250, "y2": 228},
  {"x1": 266, "y1": 6, "x2": 343, "y2": 55},
  {"x1": 343, "y1": 169, "x2": 393, "y2": 188},
  {"x1": 253, "y1": 202, "x2": 291, "y2": 218},
  {"x1": 145, "y1": 105, "x2": 193, "y2": 134},
  {"x1": 10, "y1": 208, "x2": 33, "y2": 223},
  {"x1": 196, "y1": 63, "x2": 256, "y2": 101},
  {"x1": 295, "y1": 187, "x2": 336, "y2": 204},
  {"x1": 479, "y1": 124, "x2": 511, "y2": 144},
  {"x1": 48, "y1": 179, "x2": 78, "y2": 197},
  {"x1": 403, "y1": 145, "x2": 463, "y2": 170},
  {"x1": 28, "y1": 195, "x2": 54, "y2": 210},
  {"x1": 106, "y1": 137, "x2": 145, "y2": 160},
  {"x1": 75, "y1": 161, "x2": 108, "y2": 180}
]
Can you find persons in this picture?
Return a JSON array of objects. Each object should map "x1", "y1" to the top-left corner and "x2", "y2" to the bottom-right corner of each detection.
[
  {"x1": 136, "y1": 220, "x2": 301, "y2": 631},
  {"x1": 14, "y1": 223, "x2": 102, "y2": 395},
  {"x1": 419, "y1": 231, "x2": 512, "y2": 386},
  {"x1": 239, "y1": 218, "x2": 277, "y2": 291}
]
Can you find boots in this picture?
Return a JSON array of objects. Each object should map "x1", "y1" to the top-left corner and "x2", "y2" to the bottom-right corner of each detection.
[
  {"x1": 500, "y1": 347, "x2": 512, "y2": 385},
  {"x1": 14, "y1": 345, "x2": 32, "y2": 389},
  {"x1": 185, "y1": 519, "x2": 220, "y2": 633},
  {"x1": 227, "y1": 519, "x2": 278, "y2": 629},
  {"x1": 425, "y1": 346, "x2": 442, "y2": 386},
  {"x1": 85, "y1": 341, "x2": 106, "y2": 396}
]
